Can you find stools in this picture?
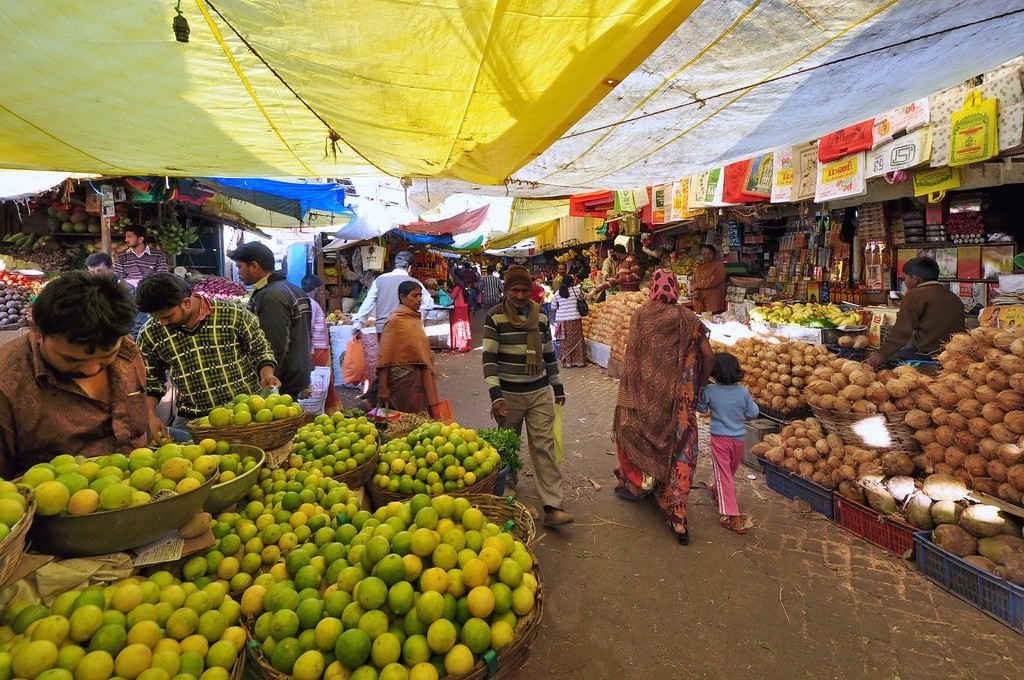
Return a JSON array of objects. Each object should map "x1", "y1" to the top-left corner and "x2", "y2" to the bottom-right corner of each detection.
[{"x1": 896, "y1": 360, "x2": 941, "y2": 369}]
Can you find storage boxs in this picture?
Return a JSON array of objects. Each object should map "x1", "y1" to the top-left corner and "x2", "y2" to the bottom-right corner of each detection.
[
  {"x1": 757, "y1": 405, "x2": 1024, "y2": 637},
  {"x1": 368, "y1": 406, "x2": 401, "y2": 430},
  {"x1": 677, "y1": 274, "x2": 691, "y2": 295},
  {"x1": 715, "y1": 238, "x2": 1014, "y2": 361}
]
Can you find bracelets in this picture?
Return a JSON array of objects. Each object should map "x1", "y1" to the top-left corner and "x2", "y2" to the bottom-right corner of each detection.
[{"x1": 699, "y1": 386, "x2": 705, "y2": 391}]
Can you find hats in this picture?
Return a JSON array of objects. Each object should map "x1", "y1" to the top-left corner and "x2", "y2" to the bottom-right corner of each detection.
[
  {"x1": 394, "y1": 251, "x2": 414, "y2": 267},
  {"x1": 504, "y1": 265, "x2": 532, "y2": 294}
]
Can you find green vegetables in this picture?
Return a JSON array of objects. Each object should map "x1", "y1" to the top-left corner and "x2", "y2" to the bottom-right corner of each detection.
[
  {"x1": 41, "y1": 273, "x2": 61, "y2": 279},
  {"x1": 477, "y1": 427, "x2": 524, "y2": 473}
]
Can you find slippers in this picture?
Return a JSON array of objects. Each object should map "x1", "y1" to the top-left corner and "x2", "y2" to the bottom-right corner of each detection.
[
  {"x1": 665, "y1": 518, "x2": 690, "y2": 545},
  {"x1": 614, "y1": 479, "x2": 635, "y2": 499}
]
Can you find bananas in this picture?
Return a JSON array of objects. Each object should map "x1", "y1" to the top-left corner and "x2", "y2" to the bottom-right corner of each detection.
[
  {"x1": 749, "y1": 301, "x2": 863, "y2": 327},
  {"x1": 672, "y1": 258, "x2": 703, "y2": 297},
  {"x1": 157, "y1": 223, "x2": 199, "y2": 255}
]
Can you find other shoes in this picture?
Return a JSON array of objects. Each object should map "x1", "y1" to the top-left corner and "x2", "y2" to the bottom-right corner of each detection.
[{"x1": 544, "y1": 509, "x2": 574, "y2": 526}]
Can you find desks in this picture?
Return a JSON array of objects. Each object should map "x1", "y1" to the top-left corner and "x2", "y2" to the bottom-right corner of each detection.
[{"x1": 424, "y1": 304, "x2": 454, "y2": 351}]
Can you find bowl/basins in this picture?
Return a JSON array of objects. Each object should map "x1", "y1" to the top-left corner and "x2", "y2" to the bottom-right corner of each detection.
[
  {"x1": 30, "y1": 466, "x2": 219, "y2": 556},
  {"x1": 201, "y1": 444, "x2": 266, "y2": 513}
]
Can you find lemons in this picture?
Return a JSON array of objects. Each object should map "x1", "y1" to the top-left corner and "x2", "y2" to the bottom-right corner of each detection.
[
  {"x1": 0, "y1": 465, "x2": 539, "y2": 679},
  {"x1": 373, "y1": 422, "x2": 500, "y2": 494},
  {"x1": 199, "y1": 393, "x2": 302, "y2": 427},
  {"x1": 0, "y1": 438, "x2": 259, "y2": 543},
  {"x1": 288, "y1": 411, "x2": 378, "y2": 476}
]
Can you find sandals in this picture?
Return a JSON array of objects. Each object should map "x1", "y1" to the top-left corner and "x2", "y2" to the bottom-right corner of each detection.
[
  {"x1": 708, "y1": 483, "x2": 720, "y2": 500},
  {"x1": 720, "y1": 517, "x2": 745, "y2": 533}
]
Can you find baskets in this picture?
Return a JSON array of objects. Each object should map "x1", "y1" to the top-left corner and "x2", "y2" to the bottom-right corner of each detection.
[
  {"x1": 754, "y1": 453, "x2": 1024, "y2": 635},
  {"x1": 331, "y1": 433, "x2": 381, "y2": 490},
  {"x1": 1, "y1": 485, "x2": 38, "y2": 586},
  {"x1": 805, "y1": 399, "x2": 925, "y2": 459},
  {"x1": 394, "y1": 492, "x2": 536, "y2": 548},
  {"x1": 239, "y1": 524, "x2": 544, "y2": 680},
  {"x1": 186, "y1": 410, "x2": 305, "y2": 450},
  {"x1": 730, "y1": 277, "x2": 764, "y2": 288},
  {"x1": 368, "y1": 457, "x2": 501, "y2": 511}
]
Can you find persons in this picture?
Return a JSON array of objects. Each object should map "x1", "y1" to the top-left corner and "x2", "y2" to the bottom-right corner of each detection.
[
  {"x1": 985, "y1": 307, "x2": 1003, "y2": 330},
  {"x1": 695, "y1": 353, "x2": 759, "y2": 533},
  {"x1": 483, "y1": 265, "x2": 574, "y2": 527},
  {"x1": 86, "y1": 254, "x2": 148, "y2": 343},
  {"x1": 114, "y1": 225, "x2": 170, "y2": 288},
  {"x1": 862, "y1": 257, "x2": 965, "y2": 374},
  {"x1": 1008, "y1": 320, "x2": 1017, "y2": 333},
  {"x1": 135, "y1": 272, "x2": 282, "y2": 450},
  {"x1": 690, "y1": 245, "x2": 727, "y2": 315},
  {"x1": 0, "y1": 269, "x2": 149, "y2": 482},
  {"x1": 301, "y1": 243, "x2": 642, "y2": 414},
  {"x1": 612, "y1": 267, "x2": 715, "y2": 548},
  {"x1": 229, "y1": 241, "x2": 314, "y2": 402}
]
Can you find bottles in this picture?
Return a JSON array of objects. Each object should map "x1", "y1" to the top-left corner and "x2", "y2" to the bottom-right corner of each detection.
[{"x1": 769, "y1": 262, "x2": 803, "y2": 282}]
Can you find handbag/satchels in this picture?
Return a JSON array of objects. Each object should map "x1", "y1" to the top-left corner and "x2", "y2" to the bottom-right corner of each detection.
[
  {"x1": 577, "y1": 299, "x2": 588, "y2": 316},
  {"x1": 296, "y1": 366, "x2": 330, "y2": 414},
  {"x1": 552, "y1": 400, "x2": 563, "y2": 471},
  {"x1": 339, "y1": 332, "x2": 378, "y2": 386},
  {"x1": 366, "y1": 398, "x2": 401, "y2": 432}
]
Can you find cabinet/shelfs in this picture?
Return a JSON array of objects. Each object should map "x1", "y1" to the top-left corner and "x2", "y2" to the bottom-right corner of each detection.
[
  {"x1": 322, "y1": 238, "x2": 342, "y2": 317},
  {"x1": 895, "y1": 242, "x2": 1018, "y2": 317},
  {"x1": 50, "y1": 196, "x2": 142, "y2": 238}
]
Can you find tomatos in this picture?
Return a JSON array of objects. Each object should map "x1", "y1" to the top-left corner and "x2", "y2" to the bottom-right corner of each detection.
[{"x1": 0, "y1": 270, "x2": 49, "y2": 290}]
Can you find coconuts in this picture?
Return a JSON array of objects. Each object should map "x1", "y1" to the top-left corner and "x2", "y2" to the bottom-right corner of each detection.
[
  {"x1": 581, "y1": 287, "x2": 651, "y2": 362},
  {"x1": 709, "y1": 326, "x2": 1024, "y2": 587}
]
